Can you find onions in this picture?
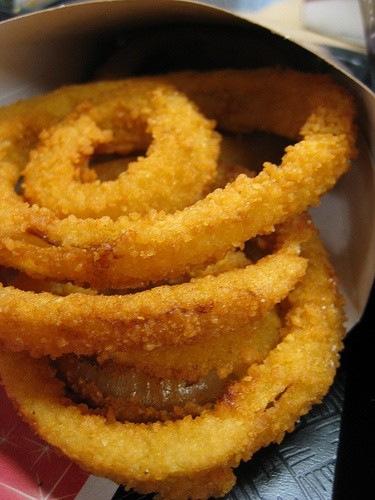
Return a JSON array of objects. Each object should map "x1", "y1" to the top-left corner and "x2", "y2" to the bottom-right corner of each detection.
[{"x1": 0, "y1": 67, "x2": 375, "y2": 500}]
